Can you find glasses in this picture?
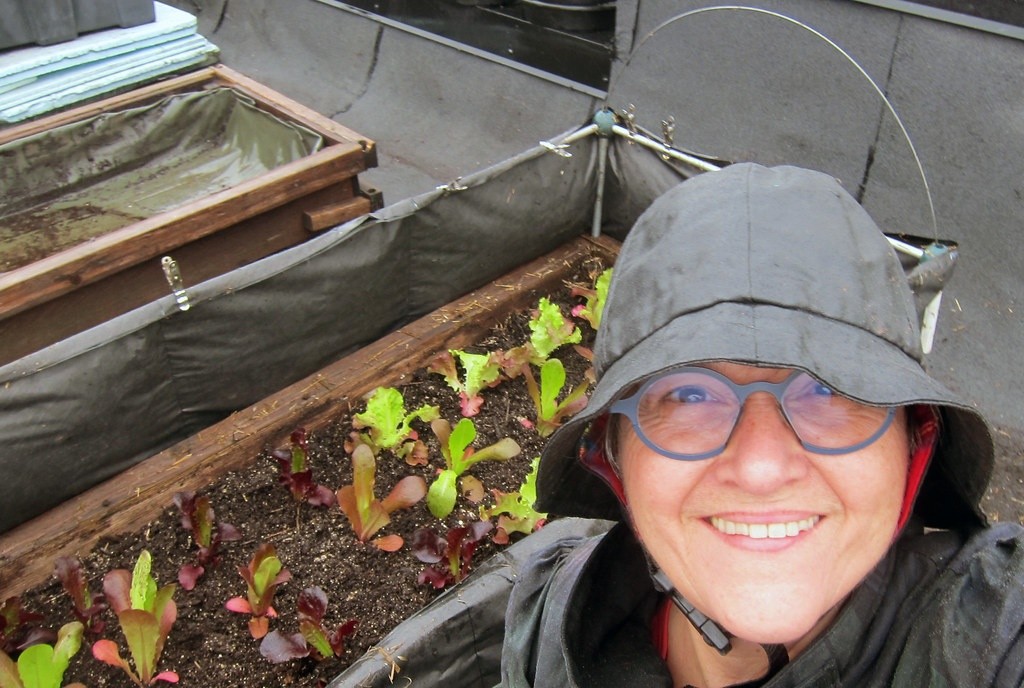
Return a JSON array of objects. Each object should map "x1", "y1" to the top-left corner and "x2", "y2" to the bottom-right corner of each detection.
[{"x1": 608, "y1": 365, "x2": 896, "y2": 461}]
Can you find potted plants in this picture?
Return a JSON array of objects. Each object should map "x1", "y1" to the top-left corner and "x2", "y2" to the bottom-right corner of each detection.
[{"x1": 2, "y1": 236, "x2": 621, "y2": 688}]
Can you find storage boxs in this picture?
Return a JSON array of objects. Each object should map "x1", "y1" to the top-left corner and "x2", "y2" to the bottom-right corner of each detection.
[
  {"x1": 1, "y1": 64, "x2": 384, "y2": 373},
  {"x1": 0, "y1": 1, "x2": 154, "y2": 52}
]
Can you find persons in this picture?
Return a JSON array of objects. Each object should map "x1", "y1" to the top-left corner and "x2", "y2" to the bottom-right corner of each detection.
[{"x1": 495, "y1": 162, "x2": 1024, "y2": 688}]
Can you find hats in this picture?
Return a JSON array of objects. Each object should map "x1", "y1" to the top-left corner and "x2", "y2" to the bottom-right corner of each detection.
[{"x1": 530, "y1": 160, "x2": 994, "y2": 533}]
